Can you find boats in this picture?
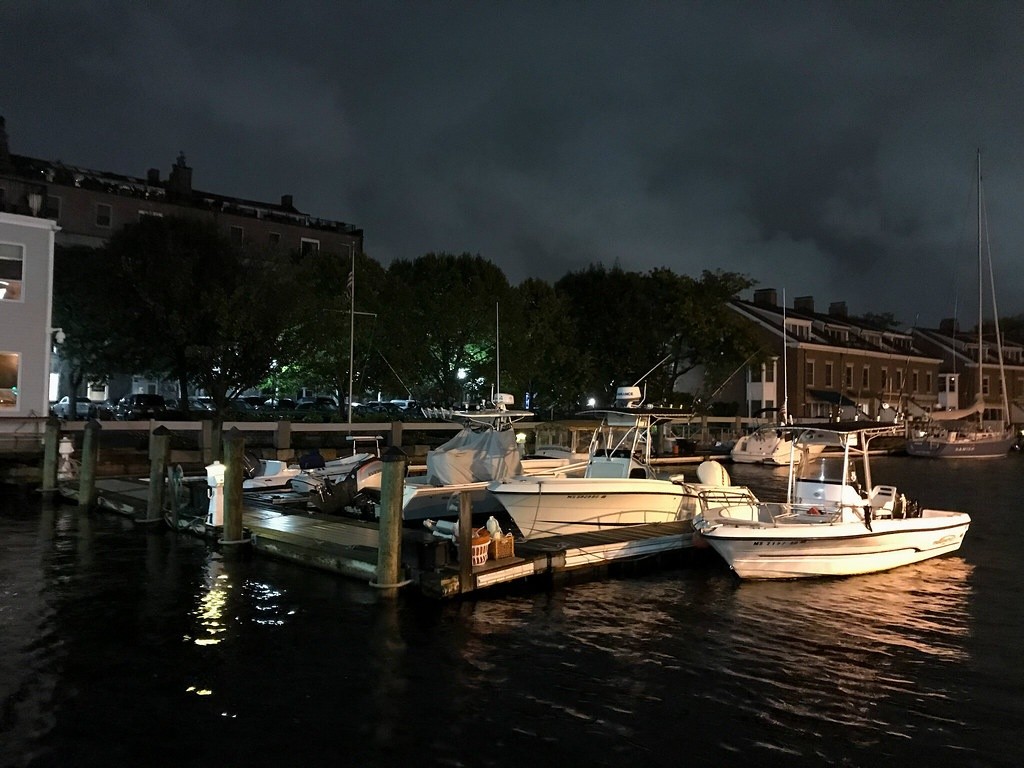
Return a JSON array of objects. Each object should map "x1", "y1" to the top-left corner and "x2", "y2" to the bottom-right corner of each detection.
[
  {"x1": 691, "y1": 422, "x2": 974, "y2": 580},
  {"x1": 206, "y1": 392, "x2": 761, "y2": 601},
  {"x1": 453, "y1": 150, "x2": 1024, "y2": 486}
]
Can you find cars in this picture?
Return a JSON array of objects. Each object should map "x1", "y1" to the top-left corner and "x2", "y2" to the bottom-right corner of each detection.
[{"x1": 42, "y1": 361, "x2": 483, "y2": 421}]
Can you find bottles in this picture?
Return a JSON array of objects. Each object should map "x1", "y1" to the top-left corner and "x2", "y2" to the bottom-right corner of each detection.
[{"x1": 486, "y1": 516, "x2": 500, "y2": 536}]
